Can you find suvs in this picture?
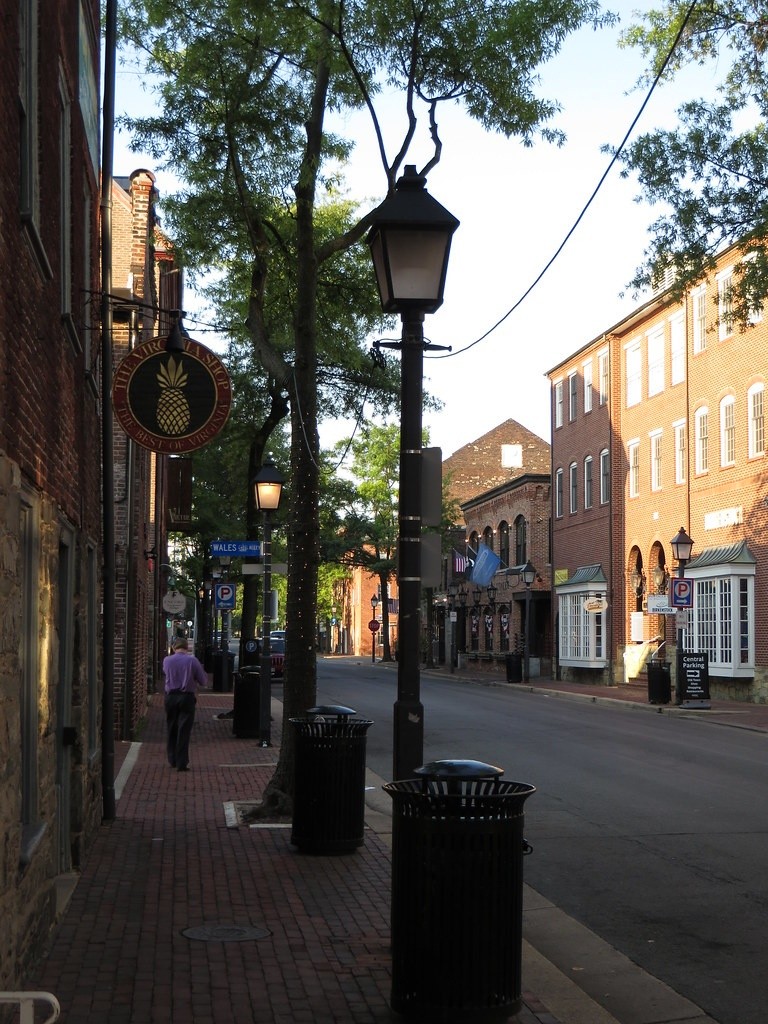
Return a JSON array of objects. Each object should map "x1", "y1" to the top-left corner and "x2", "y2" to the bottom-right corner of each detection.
[{"x1": 254, "y1": 635, "x2": 286, "y2": 679}]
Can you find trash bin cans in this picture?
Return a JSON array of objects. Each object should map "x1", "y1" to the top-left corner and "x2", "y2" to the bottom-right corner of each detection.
[
  {"x1": 210, "y1": 650, "x2": 236, "y2": 694},
  {"x1": 287, "y1": 705, "x2": 375, "y2": 850},
  {"x1": 645, "y1": 658, "x2": 672, "y2": 704},
  {"x1": 231, "y1": 666, "x2": 262, "y2": 739},
  {"x1": 380, "y1": 758, "x2": 533, "y2": 1024},
  {"x1": 505, "y1": 651, "x2": 524, "y2": 683}
]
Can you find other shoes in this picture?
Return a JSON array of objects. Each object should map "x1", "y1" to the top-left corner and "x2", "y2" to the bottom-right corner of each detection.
[
  {"x1": 171, "y1": 763, "x2": 175, "y2": 768},
  {"x1": 177, "y1": 767, "x2": 190, "y2": 772}
]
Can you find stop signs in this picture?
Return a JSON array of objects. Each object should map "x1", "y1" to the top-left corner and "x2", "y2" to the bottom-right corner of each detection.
[{"x1": 369, "y1": 621, "x2": 379, "y2": 631}]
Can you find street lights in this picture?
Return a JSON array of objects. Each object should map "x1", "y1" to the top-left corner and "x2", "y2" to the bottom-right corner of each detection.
[
  {"x1": 370, "y1": 594, "x2": 379, "y2": 661},
  {"x1": 366, "y1": 162, "x2": 459, "y2": 1019},
  {"x1": 667, "y1": 527, "x2": 694, "y2": 705},
  {"x1": 250, "y1": 458, "x2": 292, "y2": 743},
  {"x1": 518, "y1": 560, "x2": 538, "y2": 684},
  {"x1": 218, "y1": 555, "x2": 232, "y2": 695}
]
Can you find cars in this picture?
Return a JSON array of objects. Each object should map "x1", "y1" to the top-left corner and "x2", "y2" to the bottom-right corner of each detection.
[
  {"x1": 271, "y1": 631, "x2": 285, "y2": 638},
  {"x1": 213, "y1": 630, "x2": 224, "y2": 647}
]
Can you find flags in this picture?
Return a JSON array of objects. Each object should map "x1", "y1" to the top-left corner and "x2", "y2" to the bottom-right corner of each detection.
[{"x1": 454, "y1": 544, "x2": 501, "y2": 587}]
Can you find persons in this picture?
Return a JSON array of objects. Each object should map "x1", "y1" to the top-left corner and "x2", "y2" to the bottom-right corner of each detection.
[{"x1": 163, "y1": 638, "x2": 208, "y2": 772}]
